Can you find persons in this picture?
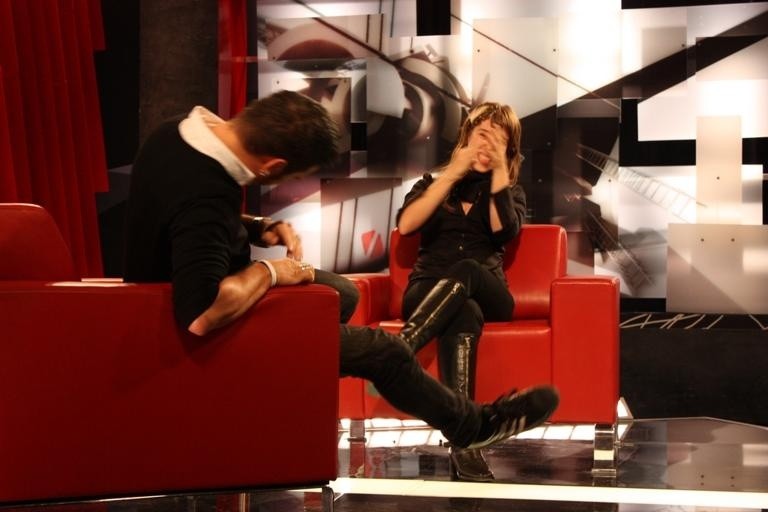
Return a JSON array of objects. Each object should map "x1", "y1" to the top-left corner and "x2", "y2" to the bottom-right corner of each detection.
[
  {"x1": 120, "y1": 90, "x2": 559, "y2": 455},
  {"x1": 394, "y1": 101, "x2": 527, "y2": 481}
]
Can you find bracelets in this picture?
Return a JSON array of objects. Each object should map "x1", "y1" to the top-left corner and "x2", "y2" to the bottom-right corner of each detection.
[{"x1": 254, "y1": 258, "x2": 277, "y2": 289}]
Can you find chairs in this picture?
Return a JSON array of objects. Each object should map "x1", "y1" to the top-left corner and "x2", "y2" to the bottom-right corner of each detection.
[
  {"x1": 0, "y1": 200, "x2": 351, "y2": 512},
  {"x1": 321, "y1": 216, "x2": 631, "y2": 470}
]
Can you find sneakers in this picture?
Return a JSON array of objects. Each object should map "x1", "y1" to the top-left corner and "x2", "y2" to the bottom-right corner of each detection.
[{"x1": 463, "y1": 381, "x2": 562, "y2": 451}]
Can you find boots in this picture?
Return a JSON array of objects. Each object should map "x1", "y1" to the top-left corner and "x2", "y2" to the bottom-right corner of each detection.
[
  {"x1": 396, "y1": 275, "x2": 471, "y2": 354},
  {"x1": 437, "y1": 334, "x2": 496, "y2": 483}
]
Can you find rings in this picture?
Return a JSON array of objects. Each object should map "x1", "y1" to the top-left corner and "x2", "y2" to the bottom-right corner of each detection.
[{"x1": 309, "y1": 270, "x2": 315, "y2": 282}]
